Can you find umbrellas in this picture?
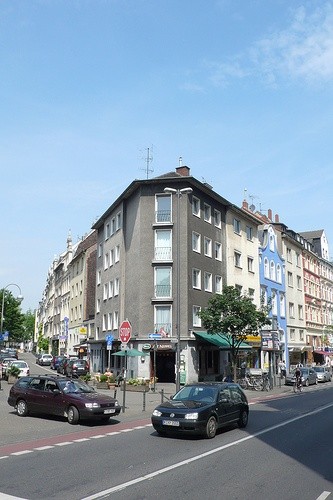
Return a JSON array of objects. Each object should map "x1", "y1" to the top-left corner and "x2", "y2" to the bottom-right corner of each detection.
[{"x1": 111, "y1": 348, "x2": 149, "y2": 357}]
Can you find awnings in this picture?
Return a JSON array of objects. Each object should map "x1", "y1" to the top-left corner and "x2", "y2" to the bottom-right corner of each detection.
[
  {"x1": 315, "y1": 350, "x2": 333, "y2": 355},
  {"x1": 236, "y1": 335, "x2": 261, "y2": 347},
  {"x1": 193, "y1": 331, "x2": 252, "y2": 349}
]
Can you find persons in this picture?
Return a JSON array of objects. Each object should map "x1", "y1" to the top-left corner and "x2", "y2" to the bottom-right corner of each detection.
[
  {"x1": 64, "y1": 381, "x2": 79, "y2": 392},
  {"x1": 213, "y1": 392, "x2": 228, "y2": 402},
  {"x1": 129, "y1": 377, "x2": 156, "y2": 391},
  {"x1": 293, "y1": 368, "x2": 302, "y2": 387},
  {"x1": 118, "y1": 368, "x2": 124, "y2": 385},
  {"x1": 280, "y1": 360, "x2": 286, "y2": 378},
  {"x1": 91, "y1": 368, "x2": 113, "y2": 382},
  {"x1": 0, "y1": 358, "x2": 30, "y2": 389}
]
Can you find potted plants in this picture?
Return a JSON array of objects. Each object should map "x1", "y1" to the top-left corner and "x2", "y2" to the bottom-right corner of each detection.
[
  {"x1": 82, "y1": 374, "x2": 115, "y2": 389},
  {"x1": 122, "y1": 378, "x2": 149, "y2": 393},
  {"x1": 7, "y1": 365, "x2": 20, "y2": 383}
]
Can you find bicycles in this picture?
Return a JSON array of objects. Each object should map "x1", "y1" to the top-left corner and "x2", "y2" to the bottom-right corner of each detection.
[
  {"x1": 238, "y1": 371, "x2": 272, "y2": 391},
  {"x1": 291, "y1": 377, "x2": 303, "y2": 393}
]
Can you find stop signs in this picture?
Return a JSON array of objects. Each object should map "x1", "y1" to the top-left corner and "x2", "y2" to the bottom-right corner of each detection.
[{"x1": 119, "y1": 320, "x2": 132, "y2": 344}]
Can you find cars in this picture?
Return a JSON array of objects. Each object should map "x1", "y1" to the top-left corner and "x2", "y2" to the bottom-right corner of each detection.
[
  {"x1": 8, "y1": 373, "x2": 122, "y2": 425},
  {"x1": 313, "y1": 367, "x2": 332, "y2": 382},
  {"x1": 1, "y1": 348, "x2": 30, "y2": 378},
  {"x1": 35, "y1": 354, "x2": 54, "y2": 365},
  {"x1": 286, "y1": 368, "x2": 318, "y2": 387},
  {"x1": 151, "y1": 381, "x2": 250, "y2": 439},
  {"x1": 50, "y1": 356, "x2": 89, "y2": 378}
]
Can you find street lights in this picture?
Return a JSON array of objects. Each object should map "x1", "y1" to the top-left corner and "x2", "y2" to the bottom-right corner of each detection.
[
  {"x1": 163, "y1": 186, "x2": 194, "y2": 395},
  {"x1": 0, "y1": 283, "x2": 23, "y2": 334}
]
